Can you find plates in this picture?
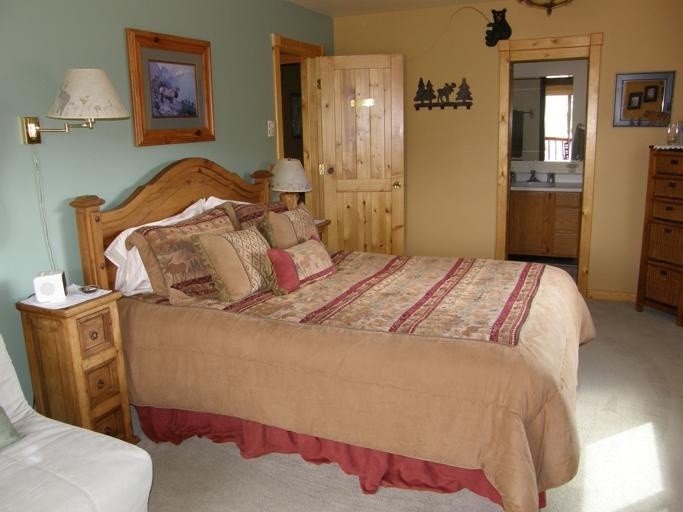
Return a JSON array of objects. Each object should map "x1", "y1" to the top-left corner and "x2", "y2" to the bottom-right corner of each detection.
[{"x1": 79, "y1": 285, "x2": 98, "y2": 294}]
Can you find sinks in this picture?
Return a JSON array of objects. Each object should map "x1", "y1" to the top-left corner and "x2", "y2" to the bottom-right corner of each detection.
[{"x1": 528, "y1": 181, "x2": 560, "y2": 187}]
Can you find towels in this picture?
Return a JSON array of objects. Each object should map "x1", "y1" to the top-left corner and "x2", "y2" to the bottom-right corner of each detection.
[
  {"x1": 571, "y1": 124, "x2": 585, "y2": 161},
  {"x1": 511, "y1": 108, "x2": 524, "y2": 158}
]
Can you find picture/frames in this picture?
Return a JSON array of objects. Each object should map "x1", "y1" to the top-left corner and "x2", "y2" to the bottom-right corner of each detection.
[
  {"x1": 126, "y1": 28, "x2": 216, "y2": 146},
  {"x1": 290, "y1": 92, "x2": 302, "y2": 138},
  {"x1": 612, "y1": 70, "x2": 675, "y2": 128}
]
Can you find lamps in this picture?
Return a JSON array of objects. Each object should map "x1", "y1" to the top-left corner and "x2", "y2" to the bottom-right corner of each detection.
[
  {"x1": 21, "y1": 69, "x2": 130, "y2": 145},
  {"x1": 270, "y1": 153, "x2": 313, "y2": 211}
]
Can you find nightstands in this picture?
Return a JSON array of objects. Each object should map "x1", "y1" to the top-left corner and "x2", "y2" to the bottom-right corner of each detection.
[
  {"x1": 312, "y1": 216, "x2": 331, "y2": 242},
  {"x1": 14, "y1": 286, "x2": 135, "y2": 445}
]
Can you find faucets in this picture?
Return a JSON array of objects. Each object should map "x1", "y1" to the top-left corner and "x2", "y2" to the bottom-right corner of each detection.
[{"x1": 547, "y1": 172, "x2": 555, "y2": 184}]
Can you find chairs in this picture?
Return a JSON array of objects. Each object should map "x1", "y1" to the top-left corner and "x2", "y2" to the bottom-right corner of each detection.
[{"x1": 0, "y1": 333, "x2": 153, "y2": 512}]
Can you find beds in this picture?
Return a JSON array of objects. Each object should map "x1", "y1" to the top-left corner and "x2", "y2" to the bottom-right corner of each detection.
[{"x1": 68, "y1": 157, "x2": 597, "y2": 512}]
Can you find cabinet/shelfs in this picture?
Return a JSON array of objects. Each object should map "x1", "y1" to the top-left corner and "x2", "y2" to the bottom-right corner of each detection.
[
  {"x1": 635, "y1": 144, "x2": 683, "y2": 326},
  {"x1": 508, "y1": 192, "x2": 582, "y2": 266}
]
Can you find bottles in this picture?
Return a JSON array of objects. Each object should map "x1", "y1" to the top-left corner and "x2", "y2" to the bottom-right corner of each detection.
[{"x1": 666, "y1": 120, "x2": 681, "y2": 146}]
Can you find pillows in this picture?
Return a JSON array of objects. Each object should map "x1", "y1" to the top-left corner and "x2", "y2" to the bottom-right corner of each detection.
[
  {"x1": 0, "y1": 406, "x2": 21, "y2": 450},
  {"x1": 103, "y1": 195, "x2": 336, "y2": 304}
]
